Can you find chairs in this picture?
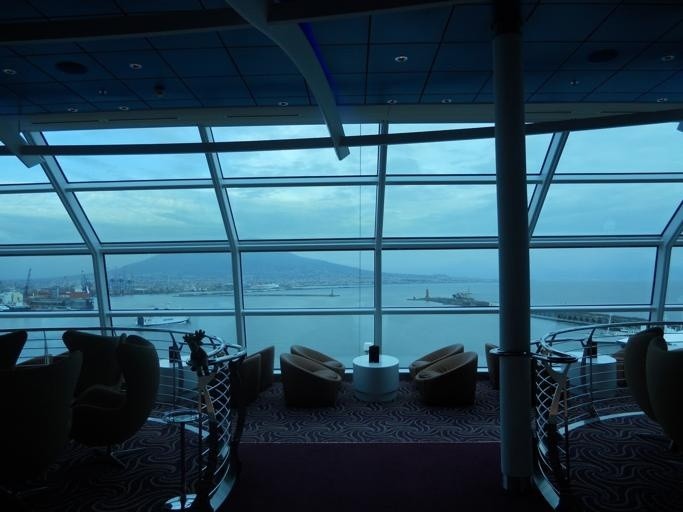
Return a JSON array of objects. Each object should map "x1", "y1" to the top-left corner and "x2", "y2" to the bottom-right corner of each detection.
[
  {"x1": 623, "y1": 328, "x2": 665, "y2": 424},
  {"x1": 409, "y1": 344, "x2": 570, "y2": 405},
  {"x1": 0, "y1": 330, "x2": 160, "y2": 512},
  {"x1": 211, "y1": 347, "x2": 345, "y2": 411},
  {"x1": 646, "y1": 337, "x2": 683, "y2": 451}
]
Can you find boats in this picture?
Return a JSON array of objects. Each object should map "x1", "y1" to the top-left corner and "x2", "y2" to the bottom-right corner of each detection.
[{"x1": 136, "y1": 314, "x2": 190, "y2": 327}]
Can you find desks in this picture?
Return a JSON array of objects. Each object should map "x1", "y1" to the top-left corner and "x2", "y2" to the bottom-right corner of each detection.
[
  {"x1": 162, "y1": 411, "x2": 203, "y2": 512},
  {"x1": 352, "y1": 355, "x2": 400, "y2": 403}
]
